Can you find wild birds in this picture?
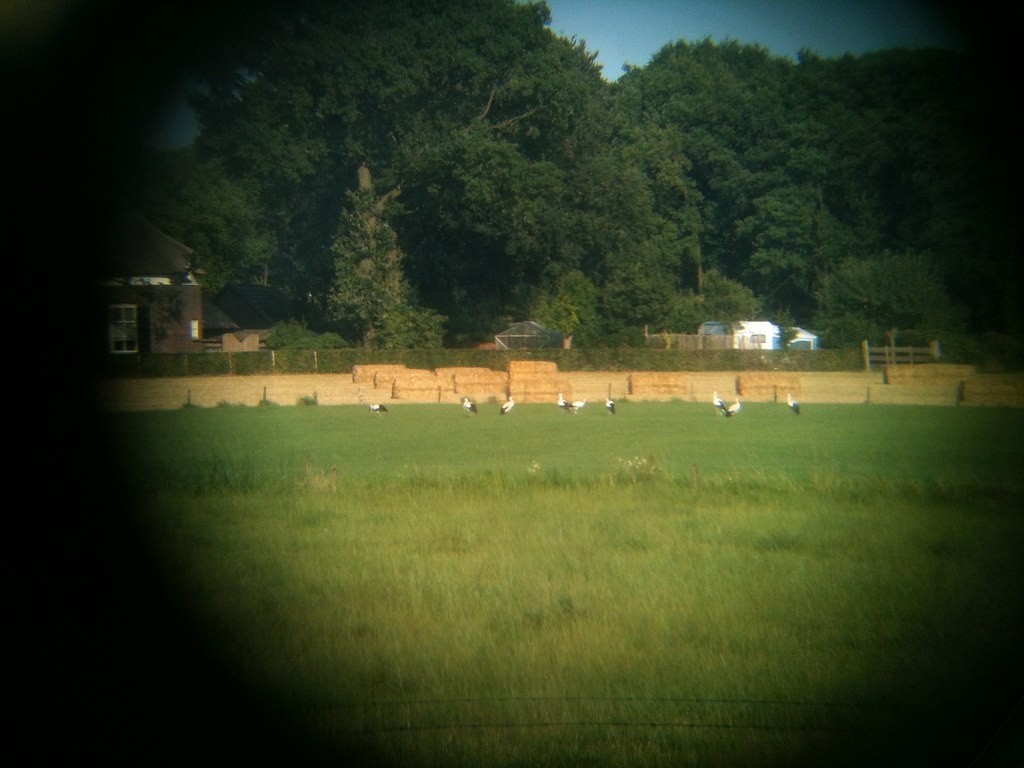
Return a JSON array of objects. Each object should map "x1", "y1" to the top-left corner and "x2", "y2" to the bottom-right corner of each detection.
[{"x1": 364, "y1": 390, "x2": 802, "y2": 417}]
